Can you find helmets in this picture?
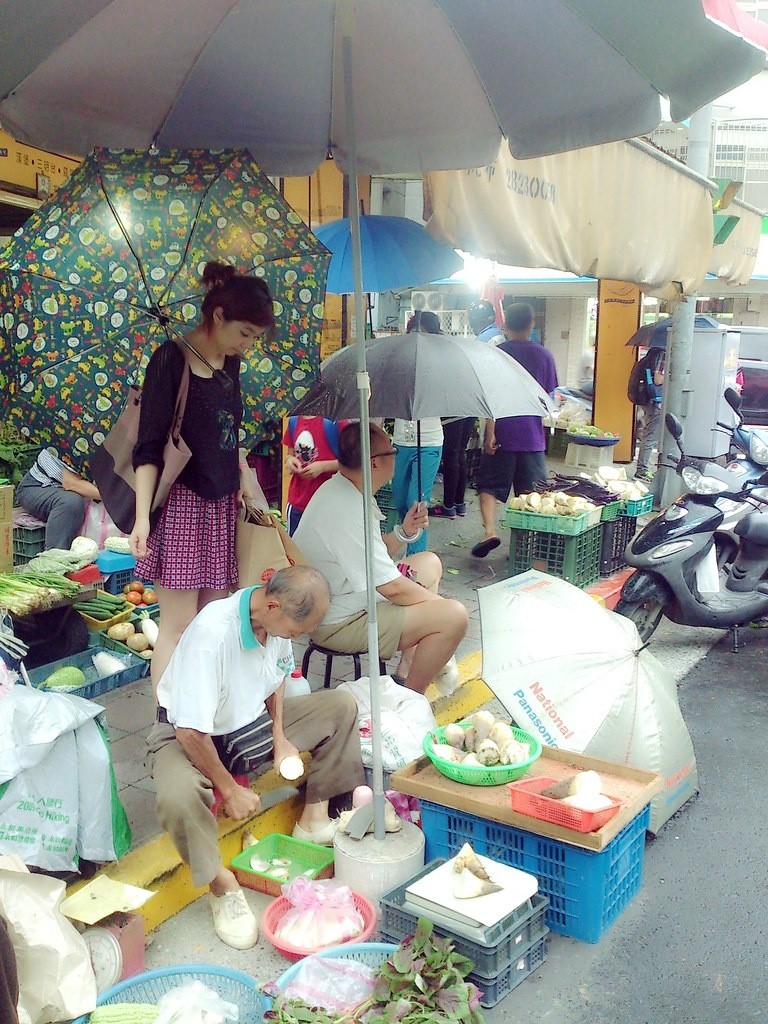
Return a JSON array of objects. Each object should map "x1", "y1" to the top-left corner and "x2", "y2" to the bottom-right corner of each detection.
[{"x1": 468, "y1": 299, "x2": 496, "y2": 330}]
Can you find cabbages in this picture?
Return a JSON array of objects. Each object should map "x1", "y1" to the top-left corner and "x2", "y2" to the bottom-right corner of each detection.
[
  {"x1": 71, "y1": 535, "x2": 100, "y2": 563},
  {"x1": 104, "y1": 536, "x2": 131, "y2": 555}
]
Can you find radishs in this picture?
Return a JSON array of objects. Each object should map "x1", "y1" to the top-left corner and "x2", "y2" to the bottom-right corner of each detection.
[{"x1": 141, "y1": 618, "x2": 159, "y2": 647}]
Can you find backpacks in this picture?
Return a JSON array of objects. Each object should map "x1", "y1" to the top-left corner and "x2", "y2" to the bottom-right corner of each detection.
[{"x1": 628, "y1": 348, "x2": 666, "y2": 405}]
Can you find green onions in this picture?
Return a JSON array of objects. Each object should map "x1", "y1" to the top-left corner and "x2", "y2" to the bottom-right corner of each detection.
[{"x1": 0, "y1": 576, "x2": 64, "y2": 615}]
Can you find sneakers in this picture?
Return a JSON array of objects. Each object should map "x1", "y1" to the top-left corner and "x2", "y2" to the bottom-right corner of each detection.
[
  {"x1": 292, "y1": 819, "x2": 339, "y2": 845},
  {"x1": 456, "y1": 503, "x2": 466, "y2": 516},
  {"x1": 209, "y1": 886, "x2": 258, "y2": 949},
  {"x1": 428, "y1": 501, "x2": 456, "y2": 518},
  {"x1": 633, "y1": 469, "x2": 655, "y2": 484}
]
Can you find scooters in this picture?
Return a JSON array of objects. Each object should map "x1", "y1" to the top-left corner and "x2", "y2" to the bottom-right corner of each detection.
[{"x1": 608, "y1": 385, "x2": 768, "y2": 655}]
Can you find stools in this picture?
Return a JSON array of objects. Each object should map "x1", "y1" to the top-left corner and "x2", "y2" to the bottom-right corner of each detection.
[{"x1": 300, "y1": 639, "x2": 385, "y2": 688}]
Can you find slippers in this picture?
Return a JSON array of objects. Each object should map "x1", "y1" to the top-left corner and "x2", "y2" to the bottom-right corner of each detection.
[{"x1": 472, "y1": 536, "x2": 500, "y2": 558}]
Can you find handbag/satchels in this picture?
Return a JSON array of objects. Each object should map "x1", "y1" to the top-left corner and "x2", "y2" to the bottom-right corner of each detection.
[
  {"x1": 217, "y1": 713, "x2": 275, "y2": 775},
  {"x1": 89, "y1": 340, "x2": 192, "y2": 534},
  {"x1": 233, "y1": 500, "x2": 309, "y2": 590},
  {"x1": 434, "y1": 655, "x2": 458, "y2": 696},
  {"x1": 157, "y1": 979, "x2": 239, "y2": 1024}
]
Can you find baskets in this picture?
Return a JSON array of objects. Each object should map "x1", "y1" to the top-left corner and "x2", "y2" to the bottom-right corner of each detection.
[
  {"x1": 99, "y1": 607, "x2": 159, "y2": 674},
  {"x1": 422, "y1": 721, "x2": 542, "y2": 786},
  {"x1": 16, "y1": 648, "x2": 146, "y2": 698},
  {"x1": 507, "y1": 776, "x2": 624, "y2": 831},
  {"x1": 77, "y1": 832, "x2": 551, "y2": 1023},
  {"x1": 115, "y1": 584, "x2": 159, "y2": 615},
  {"x1": 77, "y1": 589, "x2": 136, "y2": 630},
  {"x1": 505, "y1": 491, "x2": 654, "y2": 536},
  {"x1": 564, "y1": 430, "x2": 619, "y2": 447}
]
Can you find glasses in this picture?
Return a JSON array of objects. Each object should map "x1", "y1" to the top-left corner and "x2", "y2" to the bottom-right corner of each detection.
[{"x1": 371, "y1": 446, "x2": 398, "y2": 458}]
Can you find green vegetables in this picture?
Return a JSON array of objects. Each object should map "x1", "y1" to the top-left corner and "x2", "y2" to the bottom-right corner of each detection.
[{"x1": 261, "y1": 914, "x2": 485, "y2": 1024}]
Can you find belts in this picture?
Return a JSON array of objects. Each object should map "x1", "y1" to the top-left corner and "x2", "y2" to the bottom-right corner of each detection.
[{"x1": 156, "y1": 709, "x2": 168, "y2": 722}]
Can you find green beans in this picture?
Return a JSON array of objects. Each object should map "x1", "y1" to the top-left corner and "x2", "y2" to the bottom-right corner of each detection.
[{"x1": 4, "y1": 573, "x2": 85, "y2": 599}]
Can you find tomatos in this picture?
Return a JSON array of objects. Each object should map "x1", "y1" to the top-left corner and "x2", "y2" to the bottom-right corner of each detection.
[{"x1": 118, "y1": 580, "x2": 158, "y2": 607}]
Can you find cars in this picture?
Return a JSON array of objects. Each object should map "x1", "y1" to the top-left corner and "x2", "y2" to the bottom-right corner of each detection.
[{"x1": 720, "y1": 325, "x2": 768, "y2": 428}]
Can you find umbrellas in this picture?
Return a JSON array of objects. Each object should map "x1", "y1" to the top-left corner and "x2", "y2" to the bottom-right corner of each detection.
[
  {"x1": 623, "y1": 313, "x2": 720, "y2": 347},
  {"x1": 286, "y1": 331, "x2": 559, "y2": 544},
  {"x1": 0, "y1": 0, "x2": 768, "y2": 840},
  {"x1": 311, "y1": 198, "x2": 466, "y2": 332},
  {"x1": 0, "y1": 142, "x2": 337, "y2": 481}
]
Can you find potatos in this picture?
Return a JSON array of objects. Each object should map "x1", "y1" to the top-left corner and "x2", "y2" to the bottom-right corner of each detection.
[{"x1": 107, "y1": 622, "x2": 153, "y2": 657}]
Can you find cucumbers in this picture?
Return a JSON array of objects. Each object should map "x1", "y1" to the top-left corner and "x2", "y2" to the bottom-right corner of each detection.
[{"x1": 71, "y1": 594, "x2": 130, "y2": 621}]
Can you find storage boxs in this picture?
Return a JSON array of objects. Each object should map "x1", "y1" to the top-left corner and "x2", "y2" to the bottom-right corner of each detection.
[
  {"x1": 230, "y1": 511, "x2": 293, "y2": 594},
  {"x1": 547, "y1": 426, "x2": 570, "y2": 459},
  {"x1": 372, "y1": 473, "x2": 404, "y2": 534},
  {"x1": 379, "y1": 857, "x2": 549, "y2": 1009},
  {"x1": 420, "y1": 800, "x2": 649, "y2": 944},
  {"x1": 8, "y1": 550, "x2": 161, "y2": 699},
  {"x1": 0, "y1": 485, "x2": 13, "y2": 572},
  {"x1": 505, "y1": 494, "x2": 652, "y2": 597},
  {"x1": 507, "y1": 776, "x2": 622, "y2": 833},
  {"x1": 563, "y1": 443, "x2": 613, "y2": 472},
  {"x1": 13, "y1": 507, "x2": 47, "y2": 568}
]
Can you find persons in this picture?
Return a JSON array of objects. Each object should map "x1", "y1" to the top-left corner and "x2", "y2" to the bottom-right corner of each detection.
[
  {"x1": 290, "y1": 420, "x2": 469, "y2": 695},
  {"x1": 15, "y1": 448, "x2": 103, "y2": 552},
  {"x1": 126, "y1": 260, "x2": 275, "y2": 701},
  {"x1": 473, "y1": 302, "x2": 559, "y2": 557},
  {"x1": 141, "y1": 568, "x2": 364, "y2": 951},
  {"x1": 388, "y1": 299, "x2": 501, "y2": 558},
  {"x1": 278, "y1": 407, "x2": 349, "y2": 538},
  {"x1": 632, "y1": 345, "x2": 665, "y2": 483}
]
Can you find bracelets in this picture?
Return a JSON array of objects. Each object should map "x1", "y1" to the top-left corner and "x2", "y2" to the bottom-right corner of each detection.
[{"x1": 402, "y1": 526, "x2": 416, "y2": 538}]
